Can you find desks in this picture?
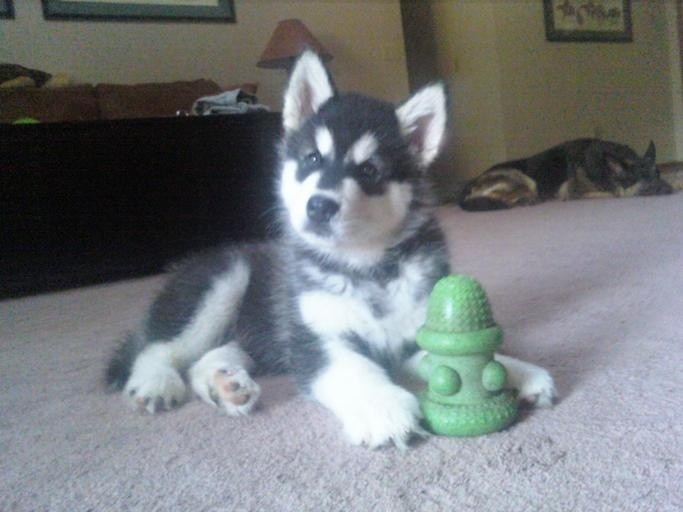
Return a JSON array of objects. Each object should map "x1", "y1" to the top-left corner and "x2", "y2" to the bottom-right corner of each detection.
[{"x1": 0, "y1": 108, "x2": 284, "y2": 299}]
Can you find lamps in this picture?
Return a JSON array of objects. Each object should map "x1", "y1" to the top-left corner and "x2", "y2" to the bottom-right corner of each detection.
[{"x1": 255, "y1": 20, "x2": 335, "y2": 103}]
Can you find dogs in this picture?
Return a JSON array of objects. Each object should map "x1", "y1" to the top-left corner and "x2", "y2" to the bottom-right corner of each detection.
[
  {"x1": 457, "y1": 136, "x2": 683, "y2": 213},
  {"x1": 99, "y1": 45, "x2": 561, "y2": 456}
]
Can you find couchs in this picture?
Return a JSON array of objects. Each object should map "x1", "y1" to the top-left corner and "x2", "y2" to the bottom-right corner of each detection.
[{"x1": 0, "y1": 76, "x2": 259, "y2": 120}]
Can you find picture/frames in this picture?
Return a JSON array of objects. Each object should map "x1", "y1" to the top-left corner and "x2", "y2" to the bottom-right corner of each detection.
[
  {"x1": 0, "y1": 0, "x2": 15, "y2": 21},
  {"x1": 543, "y1": 0, "x2": 634, "y2": 45},
  {"x1": 41, "y1": 0, "x2": 237, "y2": 24}
]
